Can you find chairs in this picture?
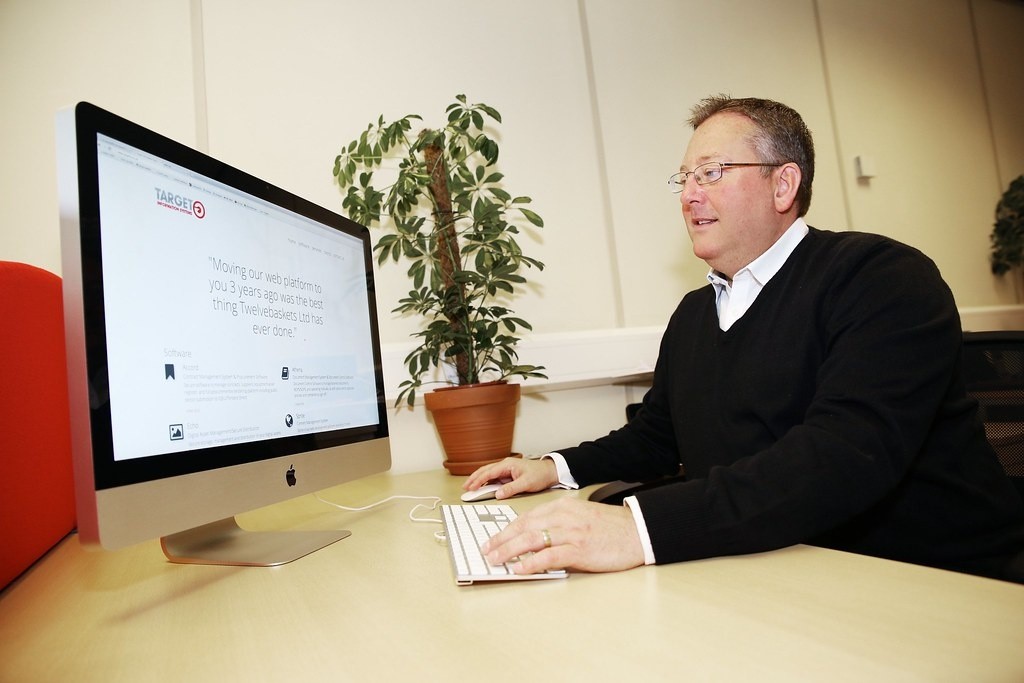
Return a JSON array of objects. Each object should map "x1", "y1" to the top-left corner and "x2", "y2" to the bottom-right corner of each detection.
[{"x1": 962, "y1": 330, "x2": 1024, "y2": 480}]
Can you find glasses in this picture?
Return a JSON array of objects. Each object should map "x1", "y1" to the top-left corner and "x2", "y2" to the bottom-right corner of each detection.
[{"x1": 668, "y1": 161, "x2": 786, "y2": 194}]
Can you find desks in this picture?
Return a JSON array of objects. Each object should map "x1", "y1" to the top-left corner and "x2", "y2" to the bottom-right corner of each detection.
[{"x1": 0, "y1": 479, "x2": 1024, "y2": 682}]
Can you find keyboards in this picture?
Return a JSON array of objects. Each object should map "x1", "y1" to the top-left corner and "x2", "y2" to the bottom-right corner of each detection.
[{"x1": 440, "y1": 505, "x2": 569, "y2": 585}]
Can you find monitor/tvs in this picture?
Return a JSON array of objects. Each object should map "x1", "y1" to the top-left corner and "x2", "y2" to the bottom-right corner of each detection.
[{"x1": 58, "y1": 100, "x2": 393, "y2": 566}]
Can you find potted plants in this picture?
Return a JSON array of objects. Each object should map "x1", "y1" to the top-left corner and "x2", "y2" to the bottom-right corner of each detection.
[{"x1": 331, "y1": 88, "x2": 545, "y2": 477}]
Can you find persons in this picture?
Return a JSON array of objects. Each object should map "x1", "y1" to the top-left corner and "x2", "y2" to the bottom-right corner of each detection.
[{"x1": 463, "y1": 94, "x2": 1023, "y2": 575}]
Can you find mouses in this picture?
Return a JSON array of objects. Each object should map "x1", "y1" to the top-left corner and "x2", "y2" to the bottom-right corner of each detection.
[{"x1": 460, "y1": 482, "x2": 506, "y2": 502}]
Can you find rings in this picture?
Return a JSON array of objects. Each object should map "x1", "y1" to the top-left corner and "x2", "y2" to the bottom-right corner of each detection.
[{"x1": 542, "y1": 529, "x2": 551, "y2": 547}]
